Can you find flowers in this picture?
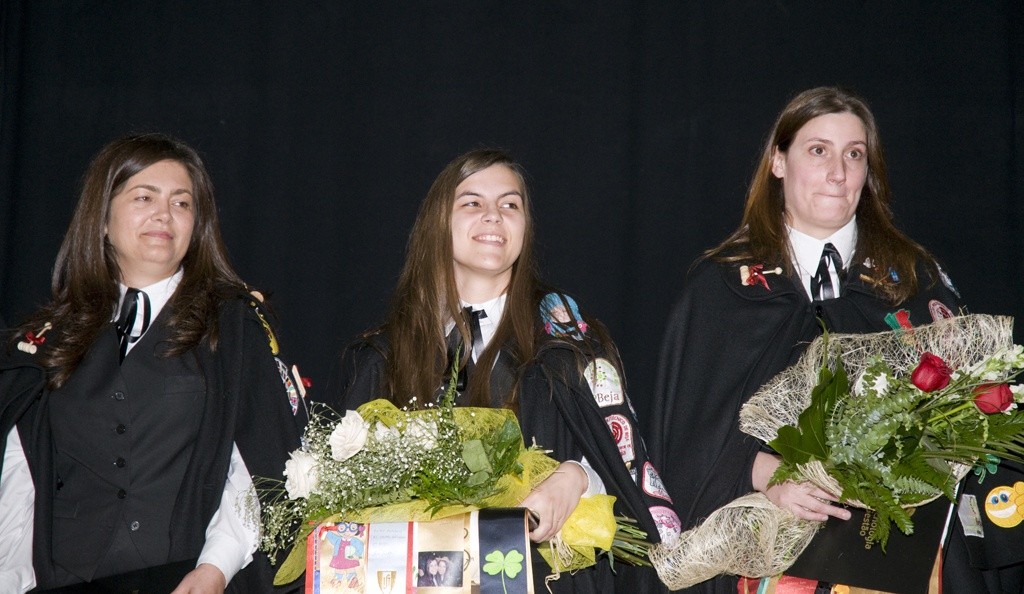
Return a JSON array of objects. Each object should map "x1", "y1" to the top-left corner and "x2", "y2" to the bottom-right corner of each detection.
[{"x1": 235, "y1": 313, "x2": 1024, "y2": 594}]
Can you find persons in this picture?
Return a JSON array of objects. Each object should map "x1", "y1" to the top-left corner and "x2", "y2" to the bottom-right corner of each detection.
[
  {"x1": 270, "y1": 147, "x2": 681, "y2": 594},
  {"x1": 0, "y1": 130, "x2": 310, "y2": 594},
  {"x1": 646, "y1": 85, "x2": 998, "y2": 594}
]
